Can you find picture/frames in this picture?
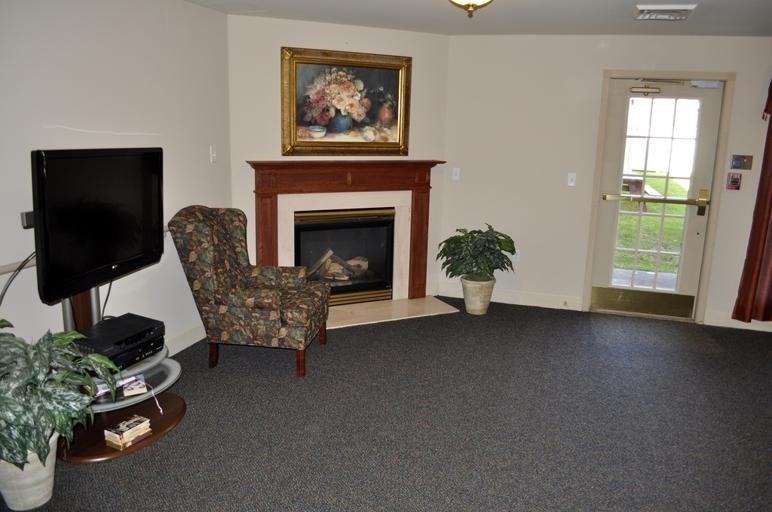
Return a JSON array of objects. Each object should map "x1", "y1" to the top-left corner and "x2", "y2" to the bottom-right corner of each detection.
[{"x1": 280, "y1": 46, "x2": 412, "y2": 157}]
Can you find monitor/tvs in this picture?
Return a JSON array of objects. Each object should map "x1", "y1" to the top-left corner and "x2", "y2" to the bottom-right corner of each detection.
[{"x1": 30, "y1": 146, "x2": 164, "y2": 306}]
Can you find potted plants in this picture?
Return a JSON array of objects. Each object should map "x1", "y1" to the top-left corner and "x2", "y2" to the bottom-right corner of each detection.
[
  {"x1": 436, "y1": 223, "x2": 515, "y2": 316},
  {"x1": 0, "y1": 319, "x2": 122, "y2": 512}
]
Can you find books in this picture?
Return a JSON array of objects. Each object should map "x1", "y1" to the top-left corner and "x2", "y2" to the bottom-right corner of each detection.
[
  {"x1": 104, "y1": 414, "x2": 153, "y2": 451},
  {"x1": 84, "y1": 373, "x2": 148, "y2": 397}
]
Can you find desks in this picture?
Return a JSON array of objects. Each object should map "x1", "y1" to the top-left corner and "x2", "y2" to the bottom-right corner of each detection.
[{"x1": 57, "y1": 344, "x2": 187, "y2": 464}]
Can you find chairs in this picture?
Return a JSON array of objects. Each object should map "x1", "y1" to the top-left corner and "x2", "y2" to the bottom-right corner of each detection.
[{"x1": 168, "y1": 205, "x2": 331, "y2": 380}]
